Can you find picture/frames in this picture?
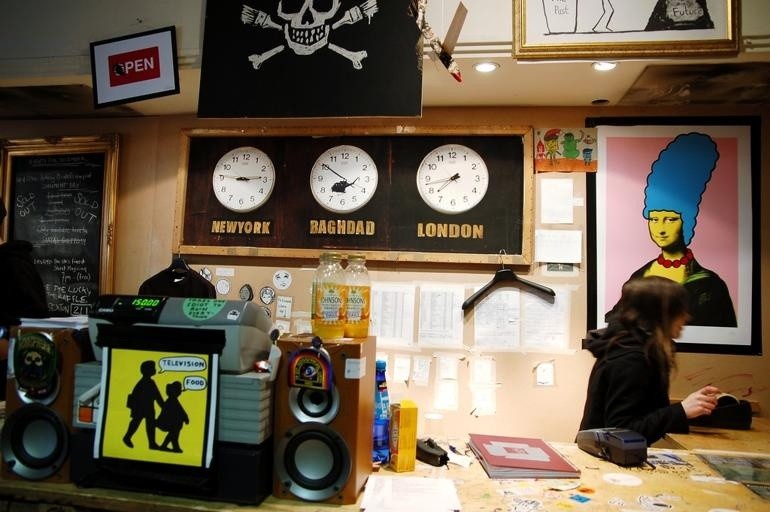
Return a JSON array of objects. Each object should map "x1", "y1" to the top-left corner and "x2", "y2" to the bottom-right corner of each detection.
[
  {"x1": 584, "y1": 114, "x2": 762, "y2": 355},
  {"x1": 171, "y1": 122, "x2": 535, "y2": 267},
  {"x1": 89, "y1": 24, "x2": 179, "y2": 110},
  {"x1": 511, "y1": 0, "x2": 740, "y2": 61}
]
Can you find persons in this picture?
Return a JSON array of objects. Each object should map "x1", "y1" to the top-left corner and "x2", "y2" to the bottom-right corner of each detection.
[
  {"x1": 607, "y1": 132, "x2": 737, "y2": 328},
  {"x1": 260, "y1": 287, "x2": 274, "y2": 305},
  {"x1": 239, "y1": 285, "x2": 253, "y2": 302},
  {"x1": 123, "y1": 361, "x2": 163, "y2": 449},
  {"x1": 0, "y1": 197, "x2": 49, "y2": 400},
  {"x1": 156, "y1": 381, "x2": 188, "y2": 452},
  {"x1": 574, "y1": 276, "x2": 722, "y2": 447}
]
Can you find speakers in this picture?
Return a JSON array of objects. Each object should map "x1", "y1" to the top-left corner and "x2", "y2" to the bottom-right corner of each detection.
[
  {"x1": 273, "y1": 331, "x2": 377, "y2": 504},
  {"x1": 0, "y1": 314, "x2": 94, "y2": 482}
]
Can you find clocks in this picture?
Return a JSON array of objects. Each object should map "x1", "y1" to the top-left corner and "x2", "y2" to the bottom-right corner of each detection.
[
  {"x1": 416, "y1": 143, "x2": 490, "y2": 215},
  {"x1": 212, "y1": 145, "x2": 277, "y2": 214},
  {"x1": 310, "y1": 143, "x2": 380, "y2": 214}
]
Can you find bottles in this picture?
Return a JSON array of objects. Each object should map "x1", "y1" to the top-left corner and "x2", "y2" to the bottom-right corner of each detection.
[
  {"x1": 372, "y1": 357, "x2": 391, "y2": 468},
  {"x1": 309, "y1": 252, "x2": 371, "y2": 346}
]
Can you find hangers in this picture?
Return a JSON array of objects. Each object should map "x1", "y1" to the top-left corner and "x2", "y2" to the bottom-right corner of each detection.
[
  {"x1": 462, "y1": 246, "x2": 555, "y2": 310},
  {"x1": 168, "y1": 241, "x2": 190, "y2": 278}
]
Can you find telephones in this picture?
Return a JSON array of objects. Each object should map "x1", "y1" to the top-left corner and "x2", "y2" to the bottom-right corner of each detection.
[{"x1": 577, "y1": 428, "x2": 656, "y2": 471}]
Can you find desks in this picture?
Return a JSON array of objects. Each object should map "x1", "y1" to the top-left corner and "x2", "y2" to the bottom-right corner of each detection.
[{"x1": 0, "y1": 392, "x2": 770, "y2": 511}]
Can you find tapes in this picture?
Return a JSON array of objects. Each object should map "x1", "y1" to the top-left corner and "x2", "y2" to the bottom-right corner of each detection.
[{"x1": 715, "y1": 393, "x2": 740, "y2": 406}]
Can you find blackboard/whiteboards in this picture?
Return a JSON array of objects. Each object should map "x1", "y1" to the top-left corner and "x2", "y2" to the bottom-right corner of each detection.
[{"x1": 2, "y1": 134, "x2": 120, "y2": 316}]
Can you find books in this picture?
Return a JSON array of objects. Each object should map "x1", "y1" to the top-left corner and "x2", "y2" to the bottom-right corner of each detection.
[{"x1": 465, "y1": 434, "x2": 581, "y2": 478}]
